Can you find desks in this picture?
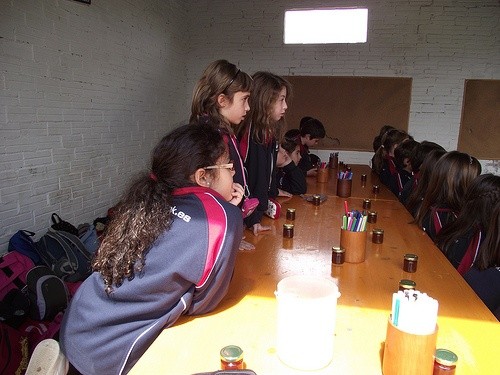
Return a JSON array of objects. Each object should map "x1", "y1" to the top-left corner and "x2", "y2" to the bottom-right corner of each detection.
[{"x1": 126, "y1": 164, "x2": 500, "y2": 375}]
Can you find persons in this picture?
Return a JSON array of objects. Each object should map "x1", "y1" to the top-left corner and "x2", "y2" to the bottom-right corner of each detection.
[
  {"x1": 284, "y1": 116, "x2": 314, "y2": 142},
  {"x1": 437, "y1": 173, "x2": 500, "y2": 284},
  {"x1": 290, "y1": 119, "x2": 325, "y2": 176},
  {"x1": 415, "y1": 151, "x2": 481, "y2": 252},
  {"x1": 375, "y1": 129, "x2": 409, "y2": 189},
  {"x1": 276, "y1": 143, "x2": 307, "y2": 198},
  {"x1": 402, "y1": 141, "x2": 445, "y2": 216},
  {"x1": 392, "y1": 141, "x2": 420, "y2": 202},
  {"x1": 234, "y1": 71, "x2": 291, "y2": 236},
  {"x1": 23, "y1": 125, "x2": 241, "y2": 375},
  {"x1": 464, "y1": 203, "x2": 500, "y2": 324},
  {"x1": 282, "y1": 140, "x2": 302, "y2": 166},
  {"x1": 372, "y1": 125, "x2": 396, "y2": 177},
  {"x1": 190, "y1": 59, "x2": 255, "y2": 251}
]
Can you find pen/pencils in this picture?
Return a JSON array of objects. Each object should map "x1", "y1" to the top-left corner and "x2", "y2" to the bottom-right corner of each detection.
[
  {"x1": 313, "y1": 164, "x2": 317, "y2": 168},
  {"x1": 392, "y1": 289, "x2": 440, "y2": 335},
  {"x1": 317, "y1": 162, "x2": 328, "y2": 168},
  {"x1": 342, "y1": 200, "x2": 368, "y2": 233},
  {"x1": 329, "y1": 152, "x2": 339, "y2": 157},
  {"x1": 337, "y1": 170, "x2": 353, "y2": 180}
]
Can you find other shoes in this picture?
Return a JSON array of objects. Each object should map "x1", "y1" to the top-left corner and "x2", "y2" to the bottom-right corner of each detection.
[{"x1": 25, "y1": 338, "x2": 70, "y2": 375}]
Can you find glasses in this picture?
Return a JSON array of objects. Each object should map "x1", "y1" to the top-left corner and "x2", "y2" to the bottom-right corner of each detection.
[{"x1": 203, "y1": 160, "x2": 236, "y2": 176}]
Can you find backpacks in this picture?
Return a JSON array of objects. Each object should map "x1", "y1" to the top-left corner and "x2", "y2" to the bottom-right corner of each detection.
[{"x1": 0, "y1": 212, "x2": 111, "y2": 330}]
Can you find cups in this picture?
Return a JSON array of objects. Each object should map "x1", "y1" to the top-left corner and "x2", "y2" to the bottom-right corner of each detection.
[
  {"x1": 340, "y1": 228, "x2": 367, "y2": 262},
  {"x1": 317, "y1": 168, "x2": 328, "y2": 182},
  {"x1": 382, "y1": 315, "x2": 439, "y2": 375},
  {"x1": 329, "y1": 157, "x2": 338, "y2": 169},
  {"x1": 274, "y1": 275, "x2": 339, "y2": 369},
  {"x1": 336, "y1": 178, "x2": 352, "y2": 197}
]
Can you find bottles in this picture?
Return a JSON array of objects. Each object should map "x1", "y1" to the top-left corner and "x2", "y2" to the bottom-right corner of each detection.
[
  {"x1": 361, "y1": 174, "x2": 367, "y2": 181},
  {"x1": 432, "y1": 348, "x2": 458, "y2": 375},
  {"x1": 372, "y1": 184, "x2": 380, "y2": 193},
  {"x1": 219, "y1": 345, "x2": 244, "y2": 371},
  {"x1": 332, "y1": 246, "x2": 346, "y2": 264},
  {"x1": 399, "y1": 279, "x2": 416, "y2": 292},
  {"x1": 363, "y1": 198, "x2": 371, "y2": 209},
  {"x1": 313, "y1": 195, "x2": 320, "y2": 206},
  {"x1": 286, "y1": 208, "x2": 296, "y2": 221},
  {"x1": 283, "y1": 224, "x2": 294, "y2": 238},
  {"x1": 403, "y1": 254, "x2": 418, "y2": 273},
  {"x1": 367, "y1": 210, "x2": 377, "y2": 223},
  {"x1": 372, "y1": 229, "x2": 384, "y2": 244}
]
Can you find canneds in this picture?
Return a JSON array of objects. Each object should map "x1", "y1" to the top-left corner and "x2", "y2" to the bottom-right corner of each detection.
[
  {"x1": 286, "y1": 208, "x2": 296, "y2": 220},
  {"x1": 332, "y1": 247, "x2": 345, "y2": 264},
  {"x1": 398, "y1": 279, "x2": 416, "y2": 291},
  {"x1": 372, "y1": 229, "x2": 384, "y2": 244},
  {"x1": 218, "y1": 346, "x2": 244, "y2": 370},
  {"x1": 432, "y1": 349, "x2": 458, "y2": 375},
  {"x1": 313, "y1": 195, "x2": 321, "y2": 206},
  {"x1": 361, "y1": 174, "x2": 367, "y2": 182},
  {"x1": 403, "y1": 254, "x2": 418, "y2": 273},
  {"x1": 363, "y1": 198, "x2": 371, "y2": 209},
  {"x1": 373, "y1": 185, "x2": 380, "y2": 194},
  {"x1": 368, "y1": 210, "x2": 376, "y2": 223},
  {"x1": 283, "y1": 224, "x2": 294, "y2": 238}
]
